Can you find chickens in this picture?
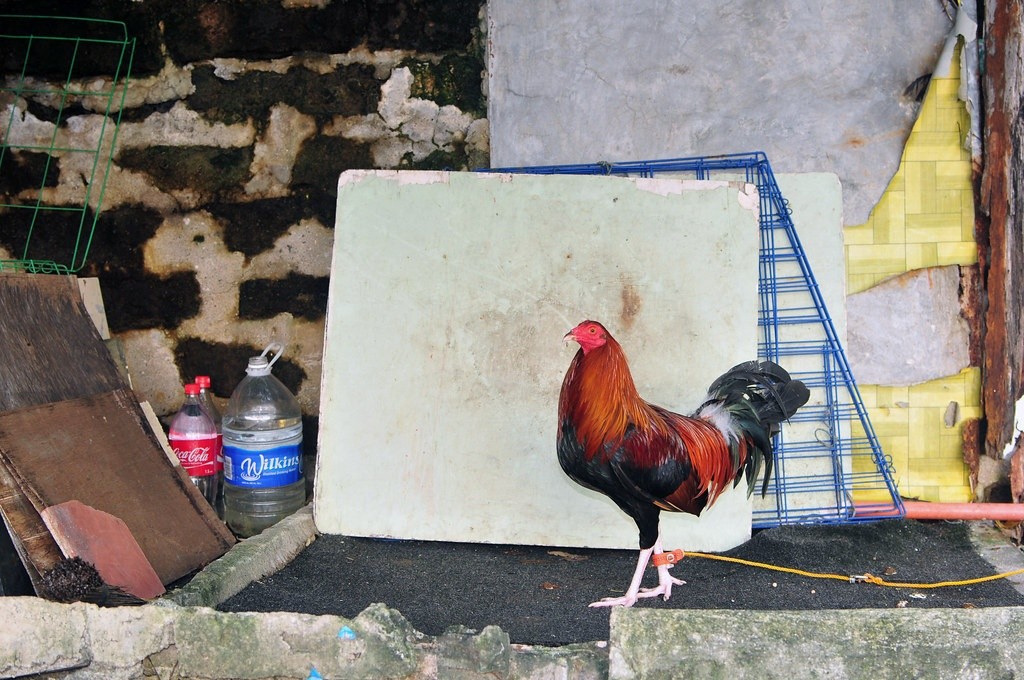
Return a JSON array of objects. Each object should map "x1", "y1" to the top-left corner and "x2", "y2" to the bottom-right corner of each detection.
[{"x1": 555, "y1": 319, "x2": 812, "y2": 608}]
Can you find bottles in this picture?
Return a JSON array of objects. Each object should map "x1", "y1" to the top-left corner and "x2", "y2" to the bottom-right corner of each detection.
[
  {"x1": 169, "y1": 382, "x2": 221, "y2": 513},
  {"x1": 195, "y1": 376, "x2": 222, "y2": 437},
  {"x1": 220, "y1": 341, "x2": 307, "y2": 537}
]
